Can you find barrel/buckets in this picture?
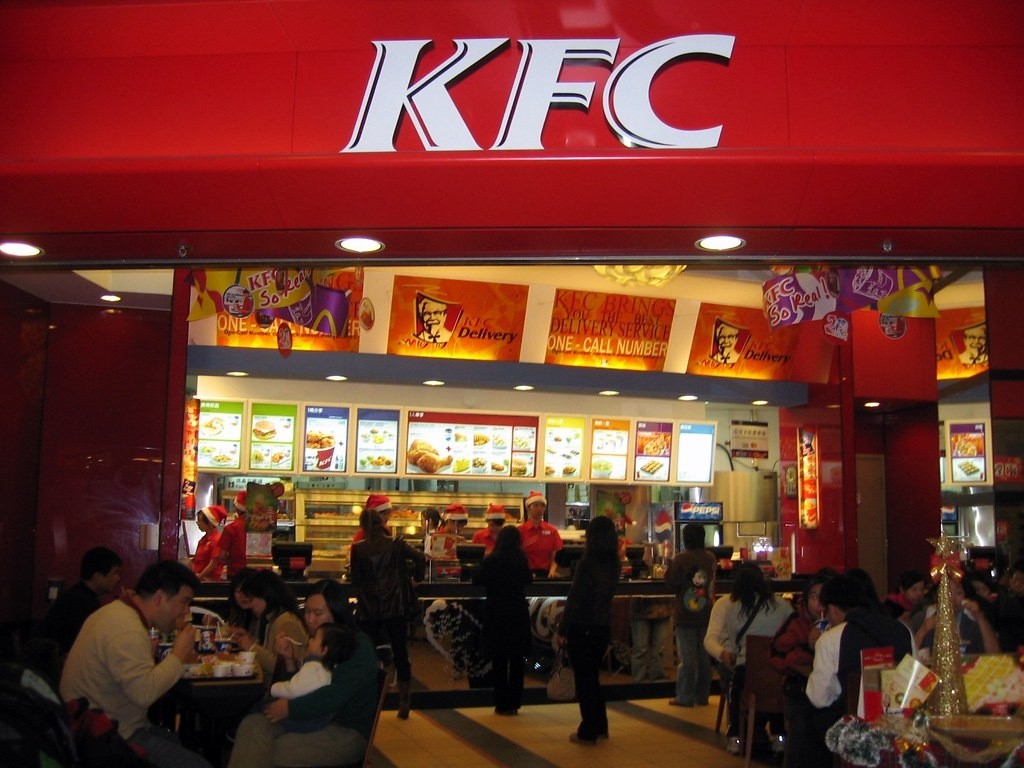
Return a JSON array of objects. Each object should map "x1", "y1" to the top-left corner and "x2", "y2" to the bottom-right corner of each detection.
[{"x1": 306, "y1": 443, "x2": 336, "y2": 471}]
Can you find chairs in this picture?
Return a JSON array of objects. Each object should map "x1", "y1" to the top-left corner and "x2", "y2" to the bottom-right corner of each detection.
[
  {"x1": 715, "y1": 626, "x2": 861, "y2": 768},
  {"x1": 354, "y1": 644, "x2": 394, "y2": 768},
  {"x1": 0, "y1": 619, "x2": 82, "y2": 768}
]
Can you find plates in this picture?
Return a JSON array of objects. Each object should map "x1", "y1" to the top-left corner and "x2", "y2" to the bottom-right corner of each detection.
[
  {"x1": 200, "y1": 420, "x2": 291, "y2": 469},
  {"x1": 644, "y1": 439, "x2": 666, "y2": 455},
  {"x1": 455, "y1": 438, "x2": 532, "y2": 474}
]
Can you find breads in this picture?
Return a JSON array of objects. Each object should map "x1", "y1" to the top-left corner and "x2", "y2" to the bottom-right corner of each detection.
[{"x1": 391, "y1": 509, "x2": 420, "y2": 518}]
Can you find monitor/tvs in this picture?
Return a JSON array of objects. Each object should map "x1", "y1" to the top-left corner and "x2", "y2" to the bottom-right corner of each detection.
[
  {"x1": 555, "y1": 545, "x2": 587, "y2": 570},
  {"x1": 272, "y1": 541, "x2": 312, "y2": 582},
  {"x1": 456, "y1": 544, "x2": 486, "y2": 560}
]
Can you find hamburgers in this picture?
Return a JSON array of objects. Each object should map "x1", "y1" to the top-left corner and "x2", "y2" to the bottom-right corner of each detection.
[
  {"x1": 253, "y1": 420, "x2": 276, "y2": 439},
  {"x1": 512, "y1": 459, "x2": 527, "y2": 475}
]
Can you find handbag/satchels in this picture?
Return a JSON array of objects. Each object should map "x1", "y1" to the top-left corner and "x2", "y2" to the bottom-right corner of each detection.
[{"x1": 546, "y1": 643, "x2": 576, "y2": 701}]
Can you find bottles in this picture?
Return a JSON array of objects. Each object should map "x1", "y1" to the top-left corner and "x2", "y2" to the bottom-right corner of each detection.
[{"x1": 335, "y1": 441, "x2": 345, "y2": 469}]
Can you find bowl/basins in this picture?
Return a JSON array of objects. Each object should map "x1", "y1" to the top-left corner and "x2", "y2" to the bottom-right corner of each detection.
[{"x1": 211, "y1": 664, "x2": 254, "y2": 677}]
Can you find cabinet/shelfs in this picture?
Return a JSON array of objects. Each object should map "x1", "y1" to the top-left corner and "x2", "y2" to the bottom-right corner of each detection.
[{"x1": 295, "y1": 489, "x2": 525, "y2": 572}]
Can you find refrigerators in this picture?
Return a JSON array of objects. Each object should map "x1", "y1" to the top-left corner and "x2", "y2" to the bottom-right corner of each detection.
[{"x1": 649, "y1": 501, "x2": 723, "y2": 569}]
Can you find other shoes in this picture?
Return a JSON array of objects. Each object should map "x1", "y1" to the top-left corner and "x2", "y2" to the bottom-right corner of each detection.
[
  {"x1": 597, "y1": 733, "x2": 609, "y2": 739},
  {"x1": 725, "y1": 726, "x2": 740, "y2": 755},
  {"x1": 396, "y1": 706, "x2": 410, "y2": 718},
  {"x1": 494, "y1": 707, "x2": 518, "y2": 716},
  {"x1": 569, "y1": 733, "x2": 598, "y2": 746},
  {"x1": 669, "y1": 697, "x2": 693, "y2": 707},
  {"x1": 764, "y1": 721, "x2": 786, "y2": 755}
]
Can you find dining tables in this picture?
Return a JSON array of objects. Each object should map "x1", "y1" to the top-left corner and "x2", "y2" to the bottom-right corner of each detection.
[{"x1": 152, "y1": 626, "x2": 264, "y2": 736}]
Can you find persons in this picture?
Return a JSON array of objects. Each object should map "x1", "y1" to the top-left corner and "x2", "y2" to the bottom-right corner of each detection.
[
  {"x1": 60, "y1": 559, "x2": 212, "y2": 768},
  {"x1": 36, "y1": 546, "x2": 123, "y2": 690},
  {"x1": 353, "y1": 492, "x2": 563, "y2": 580},
  {"x1": 703, "y1": 562, "x2": 1024, "y2": 753},
  {"x1": 558, "y1": 516, "x2": 622, "y2": 745},
  {"x1": 257, "y1": 622, "x2": 354, "y2": 733},
  {"x1": 472, "y1": 526, "x2": 533, "y2": 715},
  {"x1": 664, "y1": 522, "x2": 717, "y2": 707},
  {"x1": 351, "y1": 509, "x2": 425, "y2": 718},
  {"x1": 228, "y1": 579, "x2": 378, "y2": 768},
  {"x1": 191, "y1": 490, "x2": 310, "y2": 697},
  {"x1": 805, "y1": 573, "x2": 917, "y2": 709}
]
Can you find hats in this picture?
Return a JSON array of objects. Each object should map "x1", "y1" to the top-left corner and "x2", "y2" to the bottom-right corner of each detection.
[
  {"x1": 525, "y1": 492, "x2": 547, "y2": 508},
  {"x1": 234, "y1": 490, "x2": 247, "y2": 511},
  {"x1": 444, "y1": 504, "x2": 468, "y2": 520},
  {"x1": 365, "y1": 495, "x2": 392, "y2": 512},
  {"x1": 201, "y1": 504, "x2": 228, "y2": 527},
  {"x1": 485, "y1": 504, "x2": 506, "y2": 519}
]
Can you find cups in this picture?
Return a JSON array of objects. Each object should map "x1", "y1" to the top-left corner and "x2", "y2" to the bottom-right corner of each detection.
[
  {"x1": 721, "y1": 559, "x2": 731, "y2": 569},
  {"x1": 238, "y1": 651, "x2": 257, "y2": 665},
  {"x1": 757, "y1": 552, "x2": 767, "y2": 560},
  {"x1": 149, "y1": 625, "x2": 216, "y2": 664},
  {"x1": 214, "y1": 640, "x2": 231, "y2": 654},
  {"x1": 814, "y1": 619, "x2": 829, "y2": 633},
  {"x1": 592, "y1": 458, "x2": 613, "y2": 478},
  {"x1": 740, "y1": 547, "x2": 748, "y2": 560}
]
